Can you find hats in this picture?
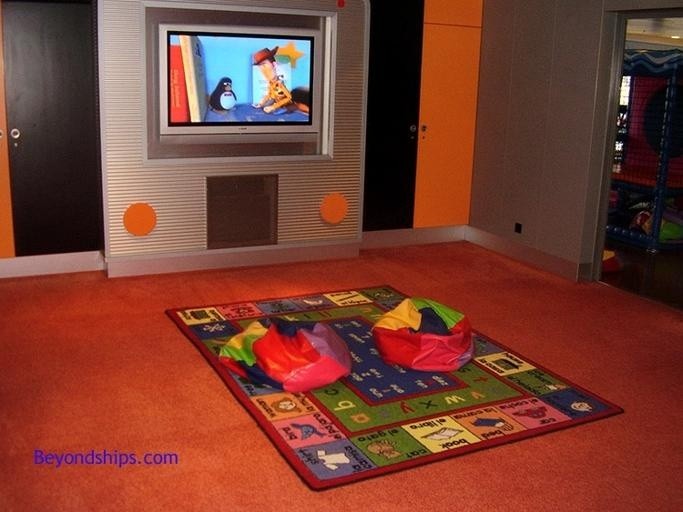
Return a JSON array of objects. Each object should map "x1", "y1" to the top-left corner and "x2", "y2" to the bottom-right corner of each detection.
[{"x1": 251, "y1": 46, "x2": 277, "y2": 65}]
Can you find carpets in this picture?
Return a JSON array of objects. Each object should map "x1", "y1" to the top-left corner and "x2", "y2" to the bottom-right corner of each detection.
[{"x1": 162, "y1": 281, "x2": 625, "y2": 493}]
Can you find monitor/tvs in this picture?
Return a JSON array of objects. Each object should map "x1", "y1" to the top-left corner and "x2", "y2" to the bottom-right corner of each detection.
[{"x1": 158, "y1": 23, "x2": 320, "y2": 134}]
